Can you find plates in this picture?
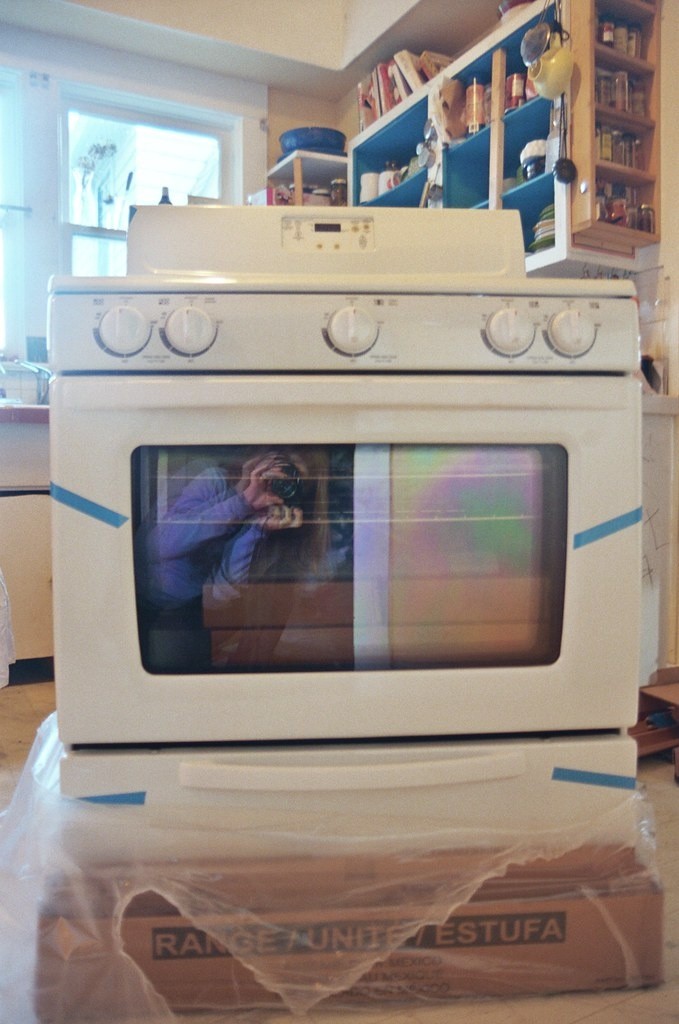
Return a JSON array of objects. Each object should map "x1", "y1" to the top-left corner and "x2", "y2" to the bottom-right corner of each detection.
[
  {"x1": 276, "y1": 145, "x2": 347, "y2": 163},
  {"x1": 502, "y1": 158, "x2": 522, "y2": 193},
  {"x1": 527, "y1": 203, "x2": 555, "y2": 250}
]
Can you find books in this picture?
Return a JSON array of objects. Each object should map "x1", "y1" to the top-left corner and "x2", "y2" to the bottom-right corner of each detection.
[{"x1": 354, "y1": 50, "x2": 456, "y2": 132}]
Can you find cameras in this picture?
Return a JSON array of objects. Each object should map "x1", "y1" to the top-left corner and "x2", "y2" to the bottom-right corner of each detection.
[{"x1": 270, "y1": 460, "x2": 306, "y2": 509}]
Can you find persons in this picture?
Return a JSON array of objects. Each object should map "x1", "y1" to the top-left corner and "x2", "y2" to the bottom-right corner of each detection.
[{"x1": 135, "y1": 447, "x2": 311, "y2": 675}]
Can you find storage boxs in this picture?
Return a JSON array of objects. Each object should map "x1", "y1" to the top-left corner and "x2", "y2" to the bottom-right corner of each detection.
[{"x1": 32, "y1": 844, "x2": 662, "y2": 1022}]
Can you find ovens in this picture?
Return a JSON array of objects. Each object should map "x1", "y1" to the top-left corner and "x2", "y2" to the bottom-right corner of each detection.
[{"x1": 42, "y1": 279, "x2": 648, "y2": 745}]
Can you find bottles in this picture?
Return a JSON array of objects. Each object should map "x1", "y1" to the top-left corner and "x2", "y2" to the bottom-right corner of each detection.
[
  {"x1": 596, "y1": 193, "x2": 654, "y2": 234},
  {"x1": 600, "y1": 12, "x2": 642, "y2": 57},
  {"x1": 378, "y1": 161, "x2": 401, "y2": 196},
  {"x1": 595, "y1": 122, "x2": 646, "y2": 169},
  {"x1": 158, "y1": 186, "x2": 172, "y2": 205},
  {"x1": 597, "y1": 70, "x2": 644, "y2": 114}
]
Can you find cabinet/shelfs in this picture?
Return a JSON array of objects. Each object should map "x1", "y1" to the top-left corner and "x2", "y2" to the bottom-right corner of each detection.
[
  {"x1": 0, "y1": 424, "x2": 55, "y2": 684},
  {"x1": 266, "y1": 0, "x2": 664, "y2": 277}
]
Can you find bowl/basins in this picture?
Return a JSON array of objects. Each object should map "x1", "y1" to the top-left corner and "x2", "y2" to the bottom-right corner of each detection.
[
  {"x1": 519, "y1": 139, "x2": 548, "y2": 180},
  {"x1": 278, "y1": 126, "x2": 347, "y2": 153}
]
